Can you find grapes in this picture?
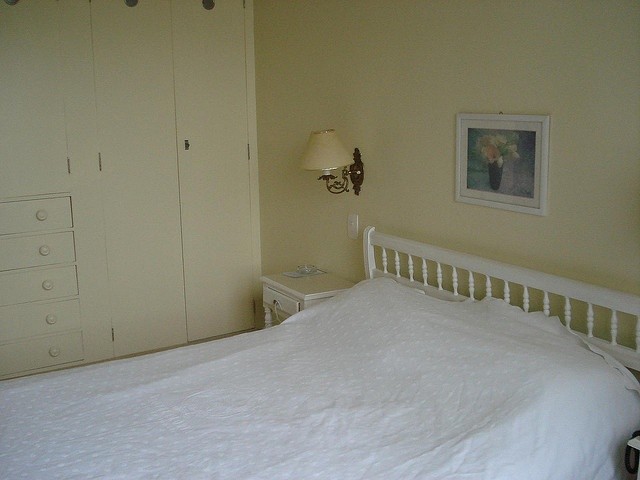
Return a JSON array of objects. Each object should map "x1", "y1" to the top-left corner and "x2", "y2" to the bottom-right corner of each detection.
[{"x1": 261, "y1": 268, "x2": 357, "y2": 325}]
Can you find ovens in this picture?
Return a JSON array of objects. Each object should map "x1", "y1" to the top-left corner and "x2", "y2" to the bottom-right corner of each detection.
[{"x1": 2, "y1": 227, "x2": 638, "y2": 480}]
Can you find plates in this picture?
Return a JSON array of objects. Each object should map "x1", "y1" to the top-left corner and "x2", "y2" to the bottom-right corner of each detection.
[
  {"x1": 88, "y1": 1, "x2": 264, "y2": 361},
  {"x1": 0, "y1": 1, "x2": 111, "y2": 380}
]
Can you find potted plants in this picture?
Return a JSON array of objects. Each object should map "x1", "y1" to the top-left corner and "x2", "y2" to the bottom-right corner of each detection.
[{"x1": 455, "y1": 112, "x2": 549, "y2": 216}]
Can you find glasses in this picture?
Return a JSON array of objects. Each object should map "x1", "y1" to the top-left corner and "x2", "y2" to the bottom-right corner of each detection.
[{"x1": 297, "y1": 129, "x2": 364, "y2": 197}]
